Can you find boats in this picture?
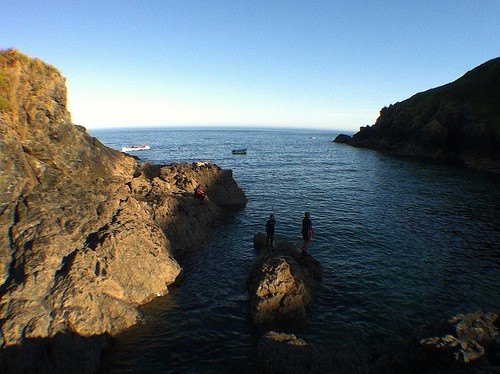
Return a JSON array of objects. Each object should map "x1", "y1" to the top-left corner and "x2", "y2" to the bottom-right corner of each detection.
[
  {"x1": 232, "y1": 149, "x2": 246, "y2": 154},
  {"x1": 122, "y1": 145, "x2": 150, "y2": 152}
]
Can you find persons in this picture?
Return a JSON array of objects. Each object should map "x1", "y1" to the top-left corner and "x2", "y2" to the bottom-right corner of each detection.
[
  {"x1": 265, "y1": 213, "x2": 276, "y2": 248},
  {"x1": 301, "y1": 212, "x2": 314, "y2": 255}
]
